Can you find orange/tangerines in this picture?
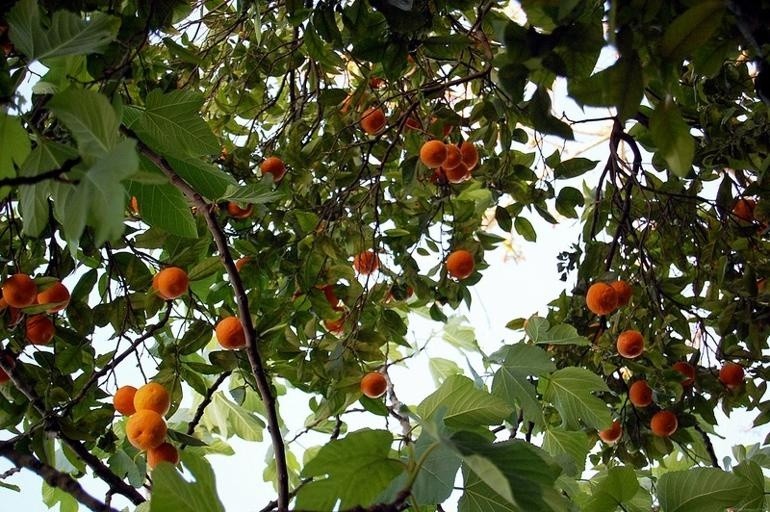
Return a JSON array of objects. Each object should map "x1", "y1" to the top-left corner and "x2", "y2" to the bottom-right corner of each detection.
[
  {"x1": 0, "y1": 76, "x2": 479, "y2": 470},
  {"x1": 585, "y1": 198, "x2": 767, "y2": 443}
]
